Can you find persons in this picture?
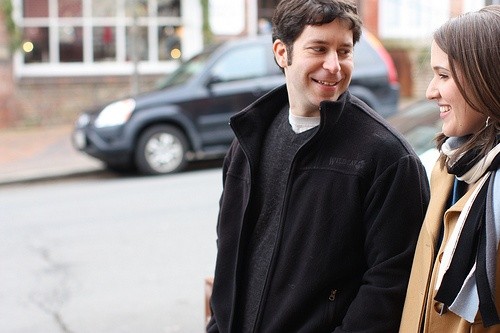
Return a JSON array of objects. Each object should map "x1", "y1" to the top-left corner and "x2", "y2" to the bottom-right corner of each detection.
[
  {"x1": 396, "y1": 5, "x2": 500, "y2": 333},
  {"x1": 206, "y1": 0, "x2": 430, "y2": 333}
]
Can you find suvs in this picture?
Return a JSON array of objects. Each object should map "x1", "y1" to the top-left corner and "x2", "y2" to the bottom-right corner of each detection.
[{"x1": 69, "y1": 34, "x2": 402, "y2": 176}]
